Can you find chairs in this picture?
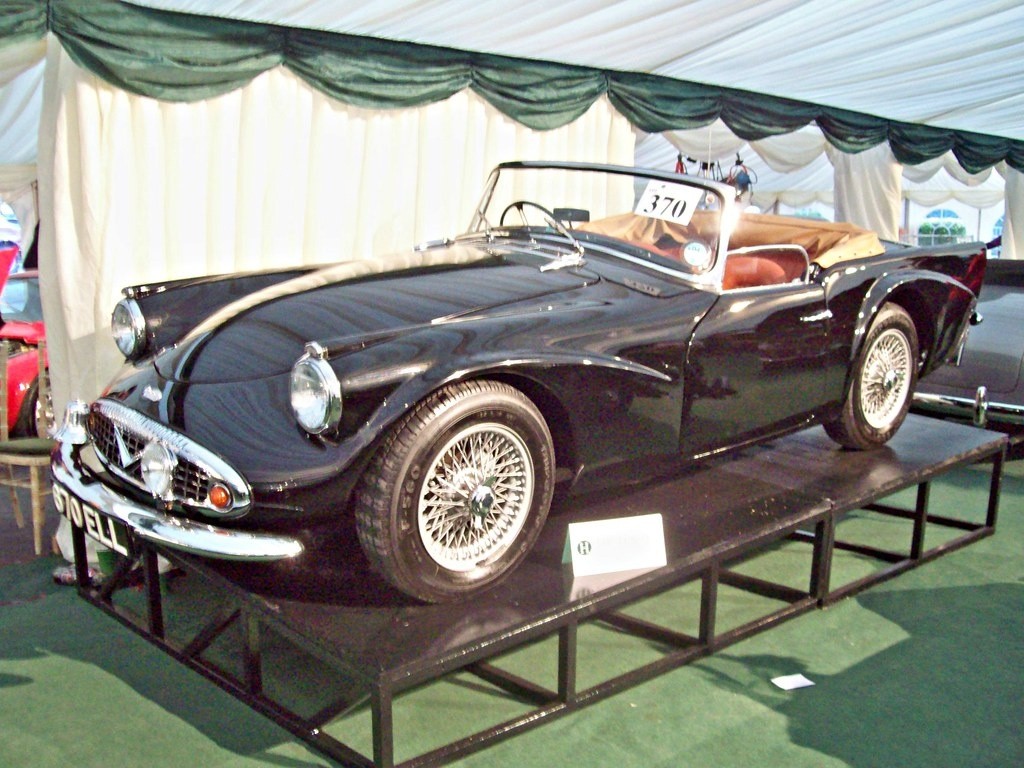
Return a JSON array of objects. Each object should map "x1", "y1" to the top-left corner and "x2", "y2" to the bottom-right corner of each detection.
[{"x1": 719, "y1": 255, "x2": 786, "y2": 288}]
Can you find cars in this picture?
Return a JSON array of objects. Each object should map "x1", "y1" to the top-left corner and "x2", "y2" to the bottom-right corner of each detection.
[
  {"x1": 0, "y1": 269, "x2": 52, "y2": 441},
  {"x1": 46, "y1": 159, "x2": 989, "y2": 607}
]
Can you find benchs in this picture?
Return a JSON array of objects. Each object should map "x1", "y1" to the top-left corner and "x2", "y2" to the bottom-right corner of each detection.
[{"x1": 588, "y1": 208, "x2": 886, "y2": 280}]
